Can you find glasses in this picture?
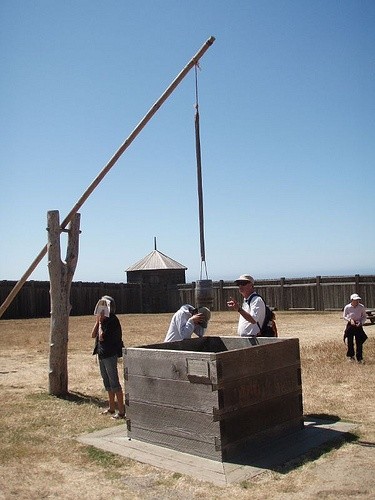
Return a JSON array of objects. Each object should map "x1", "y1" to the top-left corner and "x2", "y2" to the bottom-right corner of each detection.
[{"x1": 236, "y1": 281, "x2": 252, "y2": 286}]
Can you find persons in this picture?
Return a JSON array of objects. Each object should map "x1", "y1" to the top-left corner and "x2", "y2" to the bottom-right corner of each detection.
[
  {"x1": 342, "y1": 293, "x2": 368, "y2": 366},
  {"x1": 92, "y1": 296, "x2": 126, "y2": 420},
  {"x1": 226, "y1": 274, "x2": 266, "y2": 338},
  {"x1": 164, "y1": 304, "x2": 211, "y2": 342}
]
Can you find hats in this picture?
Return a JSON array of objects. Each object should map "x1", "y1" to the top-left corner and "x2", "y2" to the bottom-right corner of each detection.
[
  {"x1": 198, "y1": 307, "x2": 211, "y2": 329},
  {"x1": 350, "y1": 294, "x2": 361, "y2": 300},
  {"x1": 235, "y1": 275, "x2": 254, "y2": 284}
]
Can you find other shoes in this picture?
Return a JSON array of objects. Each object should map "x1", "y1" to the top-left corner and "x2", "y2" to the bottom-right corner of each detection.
[
  {"x1": 349, "y1": 356, "x2": 356, "y2": 361},
  {"x1": 99, "y1": 409, "x2": 116, "y2": 416},
  {"x1": 359, "y1": 360, "x2": 364, "y2": 364},
  {"x1": 110, "y1": 413, "x2": 126, "y2": 420}
]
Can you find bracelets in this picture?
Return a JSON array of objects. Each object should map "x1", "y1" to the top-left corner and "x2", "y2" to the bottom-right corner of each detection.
[
  {"x1": 238, "y1": 306, "x2": 242, "y2": 312},
  {"x1": 99, "y1": 322, "x2": 104, "y2": 324}
]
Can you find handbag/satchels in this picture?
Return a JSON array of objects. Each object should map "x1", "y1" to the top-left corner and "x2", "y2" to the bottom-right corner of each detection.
[
  {"x1": 248, "y1": 294, "x2": 278, "y2": 337},
  {"x1": 117, "y1": 341, "x2": 125, "y2": 358}
]
